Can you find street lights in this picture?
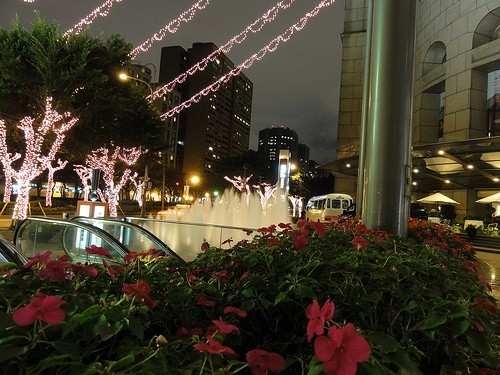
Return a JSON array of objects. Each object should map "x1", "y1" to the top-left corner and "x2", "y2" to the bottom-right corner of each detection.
[
  {"x1": 290, "y1": 163, "x2": 306, "y2": 177},
  {"x1": 182, "y1": 175, "x2": 200, "y2": 204},
  {"x1": 119, "y1": 73, "x2": 153, "y2": 219}
]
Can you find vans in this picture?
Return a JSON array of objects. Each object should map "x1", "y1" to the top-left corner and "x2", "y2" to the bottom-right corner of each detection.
[{"x1": 306, "y1": 194, "x2": 355, "y2": 225}]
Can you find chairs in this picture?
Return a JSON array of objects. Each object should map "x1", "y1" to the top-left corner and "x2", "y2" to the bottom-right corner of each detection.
[{"x1": 439, "y1": 219, "x2": 498, "y2": 236}]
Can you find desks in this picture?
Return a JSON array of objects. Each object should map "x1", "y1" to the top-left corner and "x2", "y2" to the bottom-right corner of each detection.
[{"x1": 464, "y1": 220, "x2": 484, "y2": 230}]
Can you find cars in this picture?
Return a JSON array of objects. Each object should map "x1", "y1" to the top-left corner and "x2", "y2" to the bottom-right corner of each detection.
[
  {"x1": 338, "y1": 201, "x2": 356, "y2": 223},
  {"x1": 410, "y1": 202, "x2": 429, "y2": 221}
]
